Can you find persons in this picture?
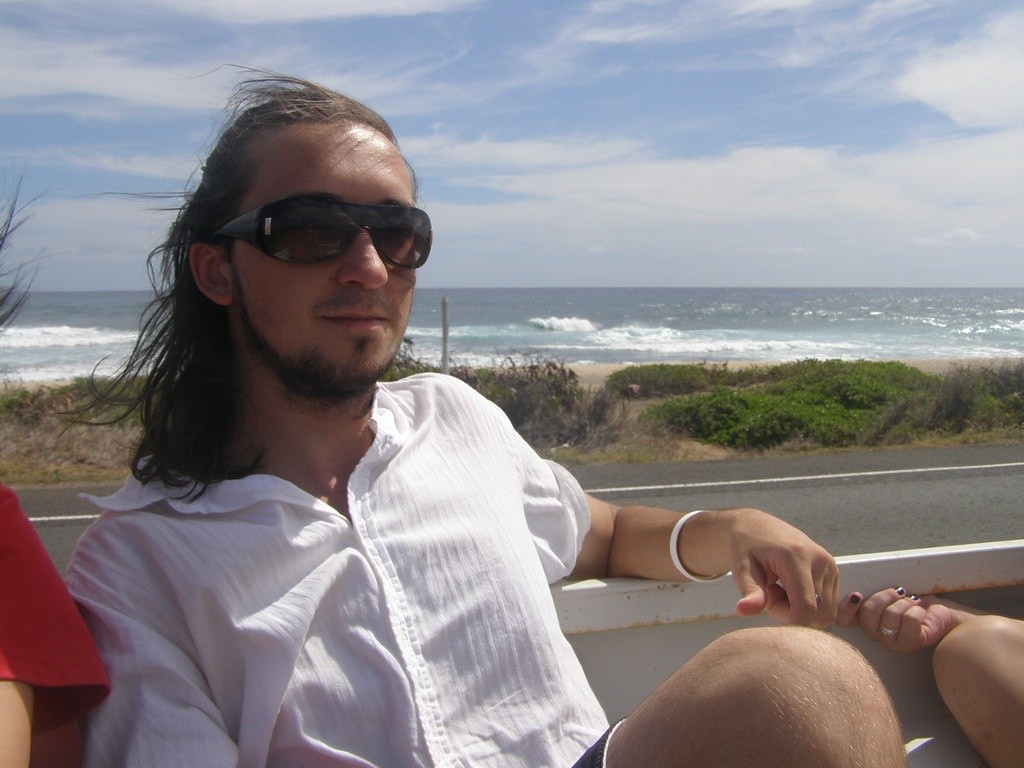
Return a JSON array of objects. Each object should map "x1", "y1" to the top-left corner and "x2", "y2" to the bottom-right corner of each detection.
[
  {"x1": 0, "y1": 482, "x2": 111, "y2": 766},
  {"x1": 69, "y1": 83, "x2": 906, "y2": 768},
  {"x1": 838, "y1": 586, "x2": 1023, "y2": 767}
]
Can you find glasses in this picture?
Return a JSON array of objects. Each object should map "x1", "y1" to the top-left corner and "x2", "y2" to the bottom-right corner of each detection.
[{"x1": 212, "y1": 196, "x2": 433, "y2": 268}]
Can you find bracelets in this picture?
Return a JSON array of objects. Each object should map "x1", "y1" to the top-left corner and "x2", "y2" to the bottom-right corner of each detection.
[{"x1": 668, "y1": 509, "x2": 735, "y2": 584}]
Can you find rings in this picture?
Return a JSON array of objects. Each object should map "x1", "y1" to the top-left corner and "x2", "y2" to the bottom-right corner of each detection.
[
  {"x1": 878, "y1": 625, "x2": 900, "y2": 637},
  {"x1": 813, "y1": 593, "x2": 822, "y2": 604}
]
